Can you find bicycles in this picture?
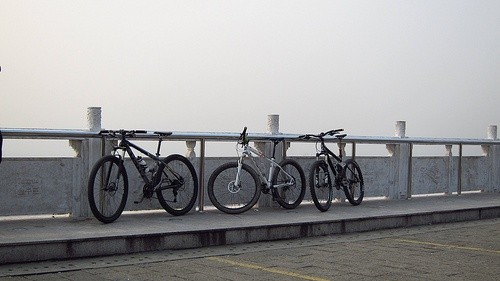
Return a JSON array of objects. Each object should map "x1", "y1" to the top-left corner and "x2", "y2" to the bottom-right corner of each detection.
[
  {"x1": 296, "y1": 128, "x2": 365, "y2": 212},
  {"x1": 88, "y1": 129, "x2": 199, "y2": 224},
  {"x1": 207, "y1": 126, "x2": 307, "y2": 215}
]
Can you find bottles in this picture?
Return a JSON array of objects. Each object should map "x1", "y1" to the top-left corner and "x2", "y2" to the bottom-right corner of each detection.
[{"x1": 137, "y1": 156, "x2": 149, "y2": 173}]
